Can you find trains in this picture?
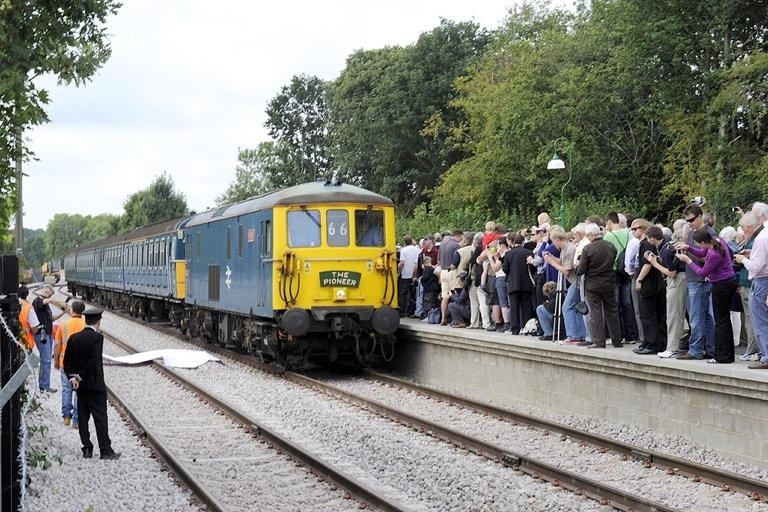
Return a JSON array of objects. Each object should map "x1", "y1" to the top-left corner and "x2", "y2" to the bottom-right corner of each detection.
[{"x1": 63, "y1": 173, "x2": 406, "y2": 375}]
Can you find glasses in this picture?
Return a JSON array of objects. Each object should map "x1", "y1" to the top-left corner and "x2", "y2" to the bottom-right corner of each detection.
[
  {"x1": 686, "y1": 215, "x2": 699, "y2": 222},
  {"x1": 631, "y1": 227, "x2": 641, "y2": 231}
]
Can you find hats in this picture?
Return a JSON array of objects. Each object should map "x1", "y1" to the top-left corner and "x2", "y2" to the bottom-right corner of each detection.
[{"x1": 81, "y1": 309, "x2": 105, "y2": 320}]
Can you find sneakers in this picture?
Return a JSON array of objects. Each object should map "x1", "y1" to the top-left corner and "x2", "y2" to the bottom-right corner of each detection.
[
  {"x1": 450, "y1": 320, "x2": 520, "y2": 334},
  {"x1": 40, "y1": 388, "x2": 57, "y2": 392},
  {"x1": 539, "y1": 335, "x2": 636, "y2": 348},
  {"x1": 409, "y1": 314, "x2": 421, "y2": 318},
  {"x1": 64, "y1": 417, "x2": 70, "y2": 425},
  {"x1": 747, "y1": 361, "x2": 768, "y2": 369},
  {"x1": 739, "y1": 353, "x2": 759, "y2": 361}
]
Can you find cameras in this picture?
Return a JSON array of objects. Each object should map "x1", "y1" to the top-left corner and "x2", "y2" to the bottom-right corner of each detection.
[{"x1": 545, "y1": 252, "x2": 551, "y2": 257}]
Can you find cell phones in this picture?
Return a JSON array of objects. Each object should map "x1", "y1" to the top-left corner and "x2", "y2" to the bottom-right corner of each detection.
[
  {"x1": 486, "y1": 245, "x2": 490, "y2": 250},
  {"x1": 677, "y1": 250, "x2": 681, "y2": 255}
]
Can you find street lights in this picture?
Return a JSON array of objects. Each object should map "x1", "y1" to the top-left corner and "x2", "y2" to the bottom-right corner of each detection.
[{"x1": 544, "y1": 135, "x2": 573, "y2": 230}]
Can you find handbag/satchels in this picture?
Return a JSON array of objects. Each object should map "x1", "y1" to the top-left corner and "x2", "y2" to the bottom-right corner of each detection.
[
  {"x1": 427, "y1": 307, "x2": 440, "y2": 323},
  {"x1": 523, "y1": 318, "x2": 538, "y2": 333}
]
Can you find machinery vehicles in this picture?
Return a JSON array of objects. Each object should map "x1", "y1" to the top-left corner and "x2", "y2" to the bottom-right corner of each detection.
[{"x1": 41, "y1": 260, "x2": 61, "y2": 283}]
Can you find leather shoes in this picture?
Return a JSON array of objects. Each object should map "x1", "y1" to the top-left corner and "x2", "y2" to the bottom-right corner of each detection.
[
  {"x1": 83, "y1": 449, "x2": 92, "y2": 458},
  {"x1": 634, "y1": 346, "x2": 719, "y2": 363},
  {"x1": 101, "y1": 453, "x2": 121, "y2": 459}
]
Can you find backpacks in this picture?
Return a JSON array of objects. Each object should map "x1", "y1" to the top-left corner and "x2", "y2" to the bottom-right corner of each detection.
[{"x1": 610, "y1": 230, "x2": 631, "y2": 285}]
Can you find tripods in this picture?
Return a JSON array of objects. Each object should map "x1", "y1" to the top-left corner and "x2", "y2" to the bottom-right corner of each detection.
[{"x1": 548, "y1": 256, "x2": 576, "y2": 347}]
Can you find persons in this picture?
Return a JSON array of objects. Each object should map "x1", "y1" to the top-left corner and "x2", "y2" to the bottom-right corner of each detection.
[
  {"x1": 62, "y1": 308, "x2": 122, "y2": 460},
  {"x1": 361, "y1": 223, "x2": 383, "y2": 248},
  {"x1": 17, "y1": 281, "x2": 87, "y2": 430}
]
